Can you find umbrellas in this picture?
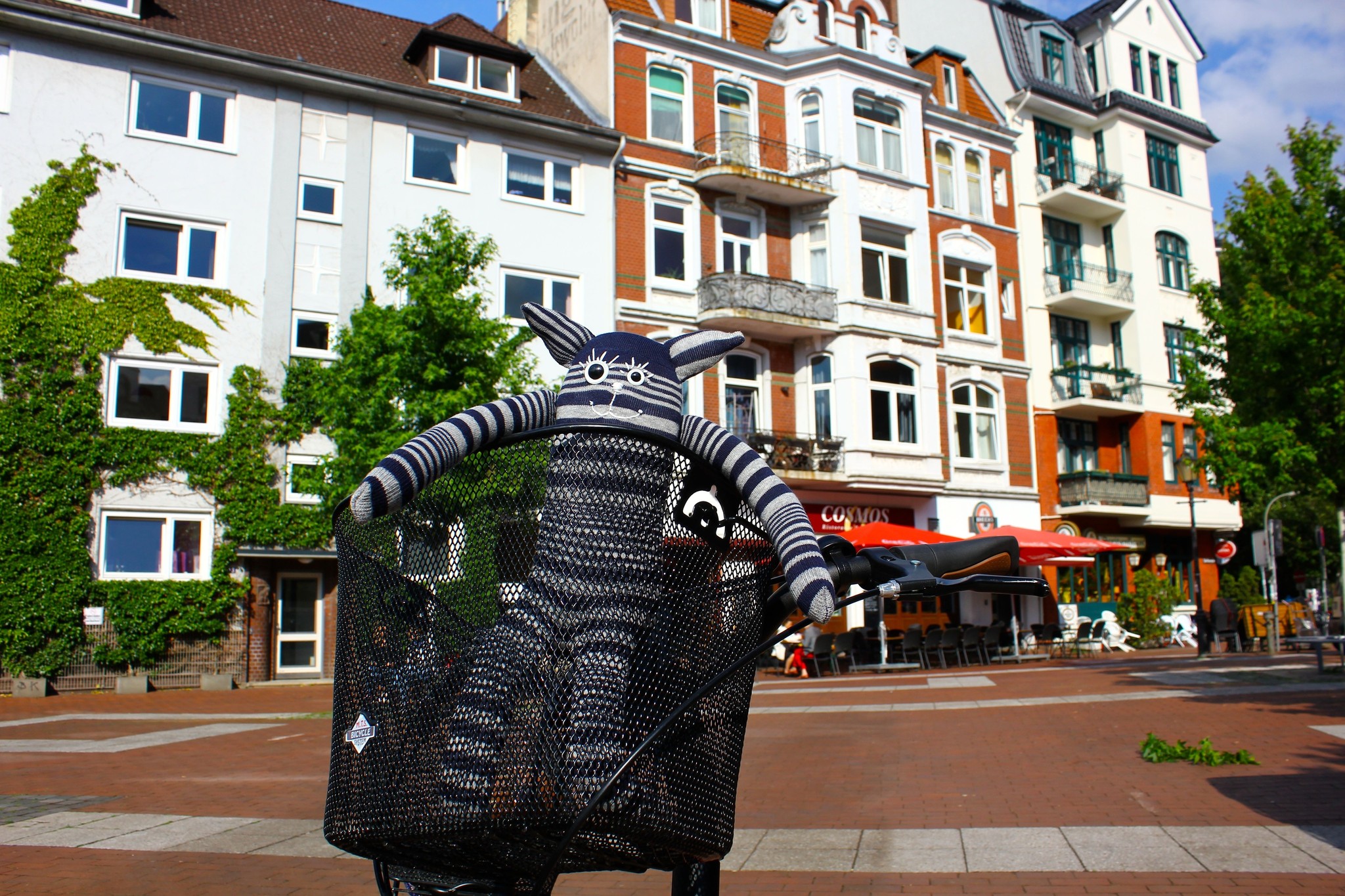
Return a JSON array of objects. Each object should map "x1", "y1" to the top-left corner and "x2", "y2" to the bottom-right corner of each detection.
[
  {"x1": 826, "y1": 518, "x2": 966, "y2": 671},
  {"x1": 962, "y1": 523, "x2": 1131, "y2": 655}
]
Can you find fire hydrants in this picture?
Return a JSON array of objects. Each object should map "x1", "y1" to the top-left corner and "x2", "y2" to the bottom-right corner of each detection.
[{"x1": 1261, "y1": 610, "x2": 1275, "y2": 651}]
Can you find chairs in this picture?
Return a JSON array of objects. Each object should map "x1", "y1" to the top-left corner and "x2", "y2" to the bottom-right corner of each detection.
[
  {"x1": 765, "y1": 616, "x2": 1143, "y2": 679},
  {"x1": 1092, "y1": 383, "x2": 1120, "y2": 403},
  {"x1": 1079, "y1": 172, "x2": 1123, "y2": 200},
  {"x1": 1157, "y1": 599, "x2": 1243, "y2": 654}
]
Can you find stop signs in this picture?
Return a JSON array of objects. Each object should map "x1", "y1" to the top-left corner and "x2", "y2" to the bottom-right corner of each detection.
[{"x1": 1215, "y1": 541, "x2": 1236, "y2": 559}]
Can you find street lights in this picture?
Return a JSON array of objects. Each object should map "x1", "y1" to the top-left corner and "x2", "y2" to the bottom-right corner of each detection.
[
  {"x1": 1262, "y1": 491, "x2": 1301, "y2": 651},
  {"x1": 1174, "y1": 444, "x2": 1212, "y2": 658}
]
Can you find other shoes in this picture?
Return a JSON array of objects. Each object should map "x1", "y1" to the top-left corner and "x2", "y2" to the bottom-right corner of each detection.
[{"x1": 794, "y1": 674, "x2": 809, "y2": 679}]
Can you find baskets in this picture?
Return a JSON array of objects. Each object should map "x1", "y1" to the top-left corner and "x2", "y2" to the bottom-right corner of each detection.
[{"x1": 323, "y1": 425, "x2": 770, "y2": 873}]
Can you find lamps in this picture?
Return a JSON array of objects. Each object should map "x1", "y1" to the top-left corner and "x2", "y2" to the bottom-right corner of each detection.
[
  {"x1": 1155, "y1": 553, "x2": 1168, "y2": 571},
  {"x1": 1127, "y1": 553, "x2": 1141, "y2": 571},
  {"x1": 782, "y1": 386, "x2": 789, "y2": 391}
]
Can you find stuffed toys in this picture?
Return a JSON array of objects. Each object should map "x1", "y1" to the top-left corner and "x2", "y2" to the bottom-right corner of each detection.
[{"x1": 350, "y1": 300, "x2": 838, "y2": 818}]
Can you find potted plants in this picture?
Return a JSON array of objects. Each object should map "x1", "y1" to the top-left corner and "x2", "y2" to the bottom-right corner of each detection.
[
  {"x1": 116, "y1": 664, "x2": 149, "y2": 692},
  {"x1": 9, "y1": 672, "x2": 50, "y2": 698},
  {"x1": 199, "y1": 669, "x2": 233, "y2": 689},
  {"x1": 1063, "y1": 357, "x2": 1078, "y2": 373}
]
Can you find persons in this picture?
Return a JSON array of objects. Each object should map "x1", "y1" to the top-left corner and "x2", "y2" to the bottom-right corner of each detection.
[
  {"x1": 770, "y1": 619, "x2": 802, "y2": 661},
  {"x1": 789, "y1": 619, "x2": 822, "y2": 679},
  {"x1": 353, "y1": 612, "x2": 442, "y2": 705}
]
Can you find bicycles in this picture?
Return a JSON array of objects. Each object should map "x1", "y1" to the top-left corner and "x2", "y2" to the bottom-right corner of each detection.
[{"x1": 324, "y1": 427, "x2": 1053, "y2": 896}]
